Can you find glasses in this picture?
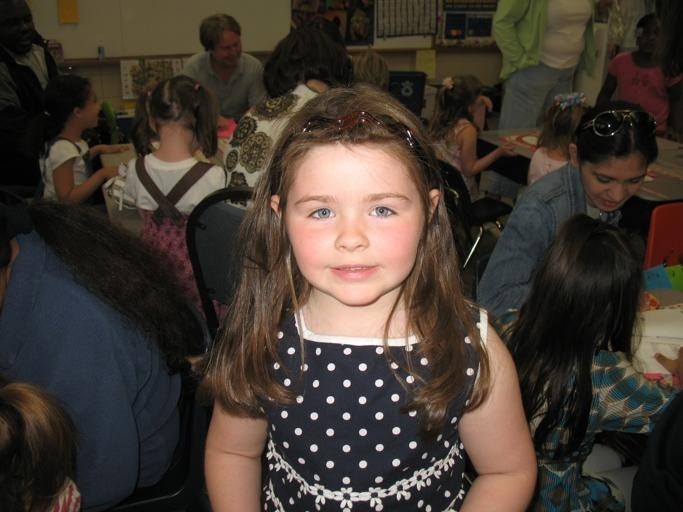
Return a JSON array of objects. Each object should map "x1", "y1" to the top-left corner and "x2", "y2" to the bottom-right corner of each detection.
[{"x1": 578, "y1": 109, "x2": 658, "y2": 138}]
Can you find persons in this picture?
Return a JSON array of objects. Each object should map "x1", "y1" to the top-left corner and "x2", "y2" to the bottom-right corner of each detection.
[{"x1": 3, "y1": 0, "x2": 682, "y2": 512}]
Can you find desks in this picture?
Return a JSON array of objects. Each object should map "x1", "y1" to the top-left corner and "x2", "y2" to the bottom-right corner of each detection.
[
  {"x1": 477, "y1": 130, "x2": 683, "y2": 232},
  {"x1": 98, "y1": 140, "x2": 157, "y2": 177}
]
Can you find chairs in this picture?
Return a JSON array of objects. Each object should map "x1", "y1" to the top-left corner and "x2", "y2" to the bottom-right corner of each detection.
[
  {"x1": 103, "y1": 102, "x2": 121, "y2": 144},
  {"x1": 111, "y1": 296, "x2": 214, "y2": 511},
  {"x1": 644, "y1": 203, "x2": 683, "y2": 270},
  {"x1": 186, "y1": 186, "x2": 287, "y2": 343},
  {"x1": 436, "y1": 158, "x2": 513, "y2": 269}
]
